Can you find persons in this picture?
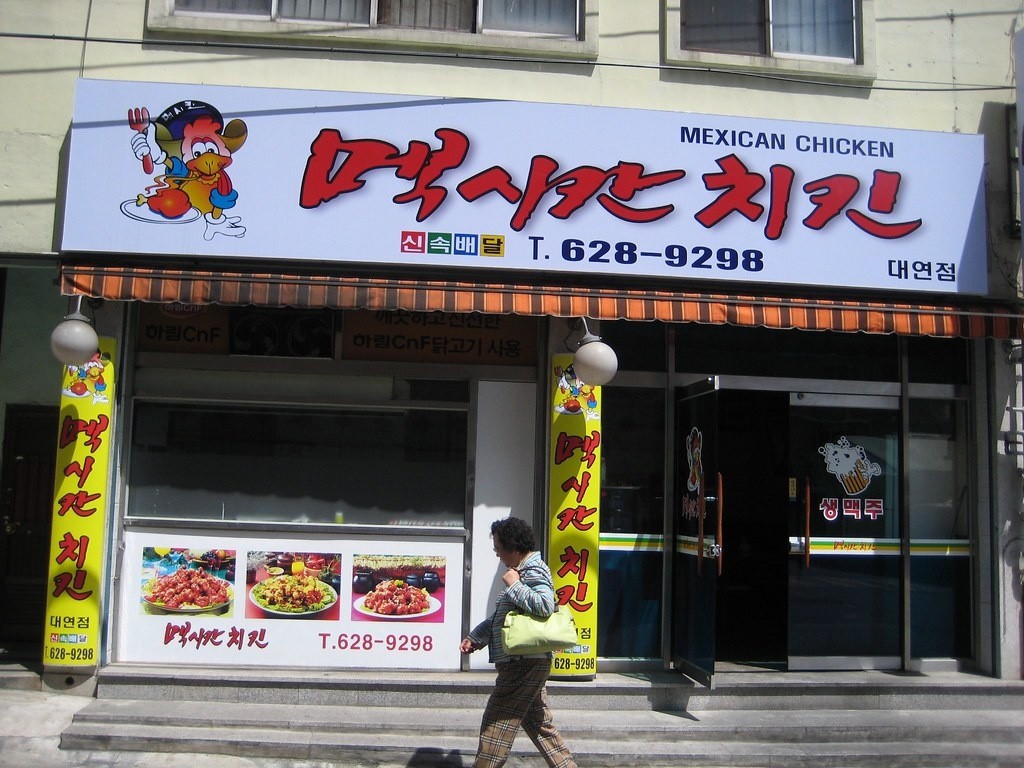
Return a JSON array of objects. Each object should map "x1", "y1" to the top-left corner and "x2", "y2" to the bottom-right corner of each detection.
[{"x1": 460, "y1": 518, "x2": 581, "y2": 768}]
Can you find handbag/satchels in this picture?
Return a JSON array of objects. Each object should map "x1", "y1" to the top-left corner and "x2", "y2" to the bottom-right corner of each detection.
[{"x1": 501, "y1": 564, "x2": 580, "y2": 657}]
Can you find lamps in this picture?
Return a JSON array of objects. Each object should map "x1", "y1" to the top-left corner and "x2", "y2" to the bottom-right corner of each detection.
[
  {"x1": 50, "y1": 294, "x2": 105, "y2": 366},
  {"x1": 568, "y1": 316, "x2": 618, "y2": 387}
]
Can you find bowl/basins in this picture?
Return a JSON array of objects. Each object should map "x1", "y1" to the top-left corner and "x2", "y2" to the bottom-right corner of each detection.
[{"x1": 267, "y1": 567, "x2": 284, "y2": 576}]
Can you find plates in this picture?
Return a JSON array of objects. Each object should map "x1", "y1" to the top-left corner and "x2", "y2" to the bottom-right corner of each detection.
[
  {"x1": 142, "y1": 574, "x2": 233, "y2": 612},
  {"x1": 353, "y1": 594, "x2": 442, "y2": 619},
  {"x1": 249, "y1": 575, "x2": 338, "y2": 615}
]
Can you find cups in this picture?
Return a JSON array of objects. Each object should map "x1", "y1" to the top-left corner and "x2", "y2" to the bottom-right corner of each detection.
[
  {"x1": 334, "y1": 511, "x2": 343, "y2": 523},
  {"x1": 290, "y1": 553, "x2": 304, "y2": 575}
]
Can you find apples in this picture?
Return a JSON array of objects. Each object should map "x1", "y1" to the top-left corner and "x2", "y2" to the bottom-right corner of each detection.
[{"x1": 216, "y1": 550, "x2": 226, "y2": 559}]
